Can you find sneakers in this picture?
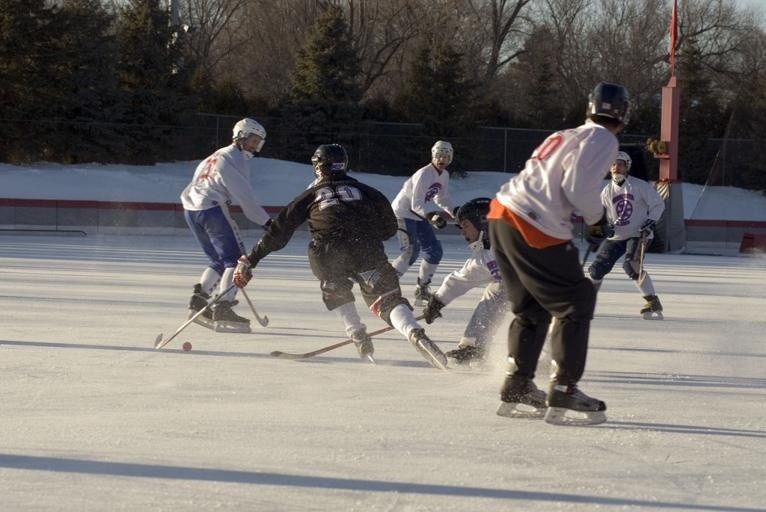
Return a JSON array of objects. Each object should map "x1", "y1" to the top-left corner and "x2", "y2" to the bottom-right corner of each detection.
[
  {"x1": 210, "y1": 298, "x2": 251, "y2": 326},
  {"x1": 188, "y1": 282, "x2": 214, "y2": 320},
  {"x1": 547, "y1": 375, "x2": 608, "y2": 412},
  {"x1": 349, "y1": 330, "x2": 375, "y2": 360},
  {"x1": 447, "y1": 342, "x2": 489, "y2": 369},
  {"x1": 638, "y1": 294, "x2": 665, "y2": 315},
  {"x1": 499, "y1": 371, "x2": 546, "y2": 408},
  {"x1": 408, "y1": 329, "x2": 448, "y2": 371}
]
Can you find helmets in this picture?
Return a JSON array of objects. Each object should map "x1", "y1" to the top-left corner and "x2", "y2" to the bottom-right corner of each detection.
[
  {"x1": 610, "y1": 151, "x2": 635, "y2": 181},
  {"x1": 587, "y1": 77, "x2": 633, "y2": 127},
  {"x1": 450, "y1": 195, "x2": 496, "y2": 242},
  {"x1": 230, "y1": 117, "x2": 268, "y2": 155},
  {"x1": 310, "y1": 141, "x2": 351, "y2": 178},
  {"x1": 430, "y1": 140, "x2": 456, "y2": 165}
]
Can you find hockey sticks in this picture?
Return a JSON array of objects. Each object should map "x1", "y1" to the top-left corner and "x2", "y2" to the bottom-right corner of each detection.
[
  {"x1": 153, "y1": 286, "x2": 238, "y2": 351},
  {"x1": 242, "y1": 286, "x2": 268, "y2": 326},
  {"x1": 272, "y1": 315, "x2": 425, "y2": 360},
  {"x1": 638, "y1": 232, "x2": 648, "y2": 287}
]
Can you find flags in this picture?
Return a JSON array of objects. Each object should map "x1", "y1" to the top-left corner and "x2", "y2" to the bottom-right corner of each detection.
[{"x1": 667, "y1": 1, "x2": 678, "y2": 67}]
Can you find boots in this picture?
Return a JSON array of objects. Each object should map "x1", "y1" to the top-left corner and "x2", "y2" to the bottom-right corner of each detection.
[{"x1": 410, "y1": 278, "x2": 441, "y2": 309}]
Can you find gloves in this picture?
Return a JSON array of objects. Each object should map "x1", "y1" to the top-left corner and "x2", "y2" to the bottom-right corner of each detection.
[
  {"x1": 584, "y1": 208, "x2": 617, "y2": 244},
  {"x1": 638, "y1": 219, "x2": 657, "y2": 240},
  {"x1": 423, "y1": 292, "x2": 445, "y2": 325},
  {"x1": 425, "y1": 209, "x2": 447, "y2": 230},
  {"x1": 231, "y1": 254, "x2": 253, "y2": 291}
]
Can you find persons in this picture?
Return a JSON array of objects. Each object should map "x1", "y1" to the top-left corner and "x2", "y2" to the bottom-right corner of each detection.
[
  {"x1": 229, "y1": 141, "x2": 451, "y2": 367},
  {"x1": 580, "y1": 148, "x2": 667, "y2": 315},
  {"x1": 422, "y1": 194, "x2": 560, "y2": 376},
  {"x1": 176, "y1": 114, "x2": 278, "y2": 329},
  {"x1": 386, "y1": 136, "x2": 455, "y2": 306},
  {"x1": 484, "y1": 78, "x2": 634, "y2": 415}
]
